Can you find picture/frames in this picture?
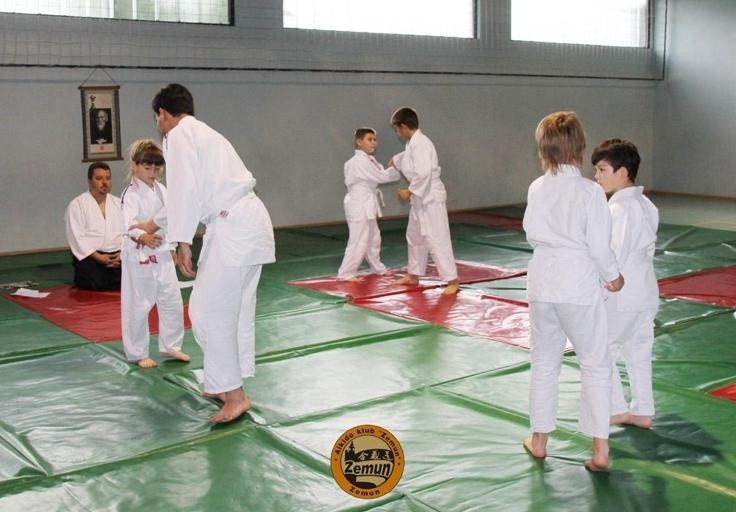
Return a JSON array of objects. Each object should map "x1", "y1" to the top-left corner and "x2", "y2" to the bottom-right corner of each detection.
[{"x1": 77, "y1": 85, "x2": 124, "y2": 164}]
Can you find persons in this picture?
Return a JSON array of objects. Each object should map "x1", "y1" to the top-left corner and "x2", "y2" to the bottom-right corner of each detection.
[
  {"x1": 116, "y1": 137, "x2": 193, "y2": 370},
  {"x1": 519, "y1": 107, "x2": 632, "y2": 473},
  {"x1": 127, "y1": 81, "x2": 280, "y2": 427},
  {"x1": 335, "y1": 126, "x2": 399, "y2": 282},
  {"x1": 583, "y1": 135, "x2": 662, "y2": 432},
  {"x1": 62, "y1": 161, "x2": 126, "y2": 292},
  {"x1": 92, "y1": 110, "x2": 112, "y2": 144},
  {"x1": 385, "y1": 105, "x2": 462, "y2": 296}
]
